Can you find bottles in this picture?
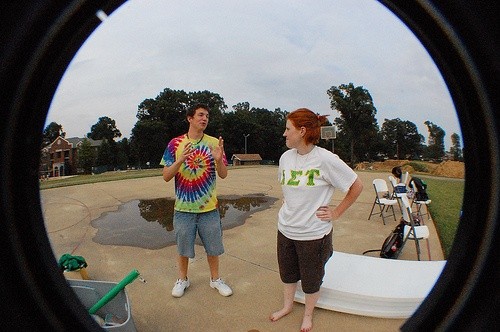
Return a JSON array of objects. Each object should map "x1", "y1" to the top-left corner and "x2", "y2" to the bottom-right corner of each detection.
[{"x1": 387, "y1": 242, "x2": 398, "y2": 257}]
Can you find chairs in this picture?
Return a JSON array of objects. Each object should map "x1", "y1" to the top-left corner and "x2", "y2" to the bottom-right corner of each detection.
[{"x1": 367, "y1": 172, "x2": 432, "y2": 260}]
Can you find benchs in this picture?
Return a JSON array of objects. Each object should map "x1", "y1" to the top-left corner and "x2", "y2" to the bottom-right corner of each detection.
[{"x1": 296, "y1": 251, "x2": 447, "y2": 320}]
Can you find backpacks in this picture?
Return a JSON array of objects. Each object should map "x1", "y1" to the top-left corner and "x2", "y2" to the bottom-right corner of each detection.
[{"x1": 362, "y1": 220, "x2": 411, "y2": 259}]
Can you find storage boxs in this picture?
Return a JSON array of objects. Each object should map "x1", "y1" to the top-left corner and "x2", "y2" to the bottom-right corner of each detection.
[{"x1": 67, "y1": 279, "x2": 138, "y2": 332}]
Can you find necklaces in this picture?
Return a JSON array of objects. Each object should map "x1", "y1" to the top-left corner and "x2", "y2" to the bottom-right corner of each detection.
[
  {"x1": 185, "y1": 129, "x2": 205, "y2": 144},
  {"x1": 294, "y1": 145, "x2": 315, "y2": 170}
]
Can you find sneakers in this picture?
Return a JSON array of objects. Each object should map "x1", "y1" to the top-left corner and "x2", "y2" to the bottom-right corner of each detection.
[
  {"x1": 209, "y1": 276, "x2": 233, "y2": 297},
  {"x1": 171, "y1": 276, "x2": 191, "y2": 298}
]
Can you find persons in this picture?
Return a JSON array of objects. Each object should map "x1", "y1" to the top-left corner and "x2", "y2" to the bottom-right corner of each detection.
[
  {"x1": 159, "y1": 103, "x2": 233, "y2": 296},
  {"x1": 268, "y1": 108, "x2": 363, "y2": 332}
]
